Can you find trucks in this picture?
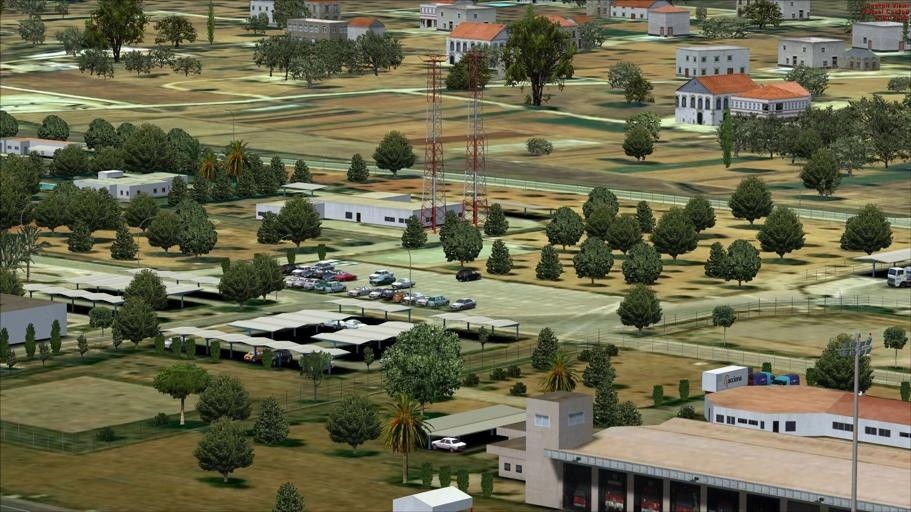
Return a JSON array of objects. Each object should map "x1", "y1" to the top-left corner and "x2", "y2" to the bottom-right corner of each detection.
[
  {"x1": 753, "y1": 372, "x2": 776, "y2": 385},
  {"x1": 702, "y1": 365, "x2": 754, "y2": 395},
  {"x1": 773, "y1": 374, "x2": 799, "y2": 385},
  {"x1": 887, "y1": 266, "x2": 911, "y2": 288},
  {"x1": 572, "y1": 470, "x2": 699, "y2": 512}
]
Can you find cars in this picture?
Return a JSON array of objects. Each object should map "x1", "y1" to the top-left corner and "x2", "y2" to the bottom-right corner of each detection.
[
  {"x1": 244, "y1": 348, "x2": 293, "y2": 366},
  {"x1": 432, "y1": 437, "x2": 467, "y2": 453},
  {"x1": 284, "y1": 261, "x2": 482, "y2": 312},
  {"x1": 165, "y1": 336, "x2": 189, "y2": 351},
  {"x1": 323, "y1": 319, "x2": 367, "y2": 329}
]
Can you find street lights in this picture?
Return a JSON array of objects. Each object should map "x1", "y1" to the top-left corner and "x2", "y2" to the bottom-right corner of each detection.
[{"x1": 835, "y1": 333, "x2": 873, "y2": 512}]
